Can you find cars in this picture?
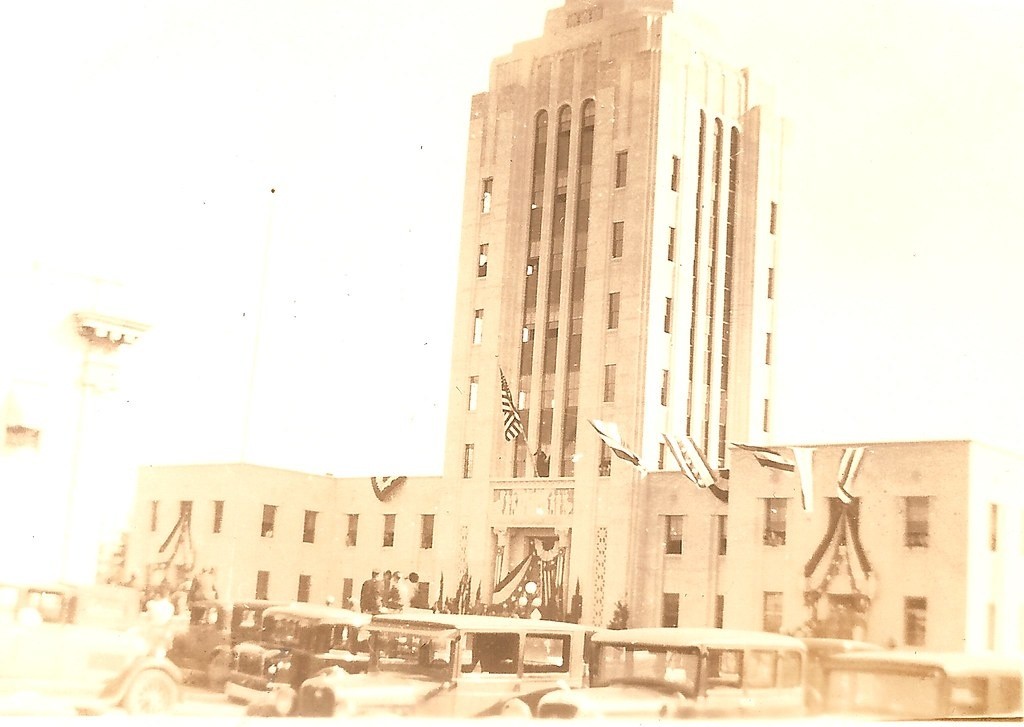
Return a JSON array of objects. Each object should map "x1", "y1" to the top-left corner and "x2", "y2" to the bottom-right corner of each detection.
[{"x1": 0, "y1": 582, "x2": 1024, "y2": 718}]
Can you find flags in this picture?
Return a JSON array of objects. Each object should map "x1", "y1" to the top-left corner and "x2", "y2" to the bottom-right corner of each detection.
[
  {"x1": 732, "y1": 442, "x2": 794, "y2": 472},
  {"x1": 498, "y1": 368, "x2": 523, "y2": 441},
  {"x1": 792, "y1": 447, "x2": 814, "y2": 511},
  {"x1": 836, "y1": 448, "x2": 865, "y2": 505},
  {"x1": 664, "y1": 433, "x2": 717, "y2": 490},
  {"x1": 588, "y1": 421, "x2": 648, "y2": 479}
]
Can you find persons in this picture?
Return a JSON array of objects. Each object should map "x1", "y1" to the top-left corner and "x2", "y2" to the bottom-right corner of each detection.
[{"x1": 360, "y1": 571, "x2": 421, "y2": 616}]
